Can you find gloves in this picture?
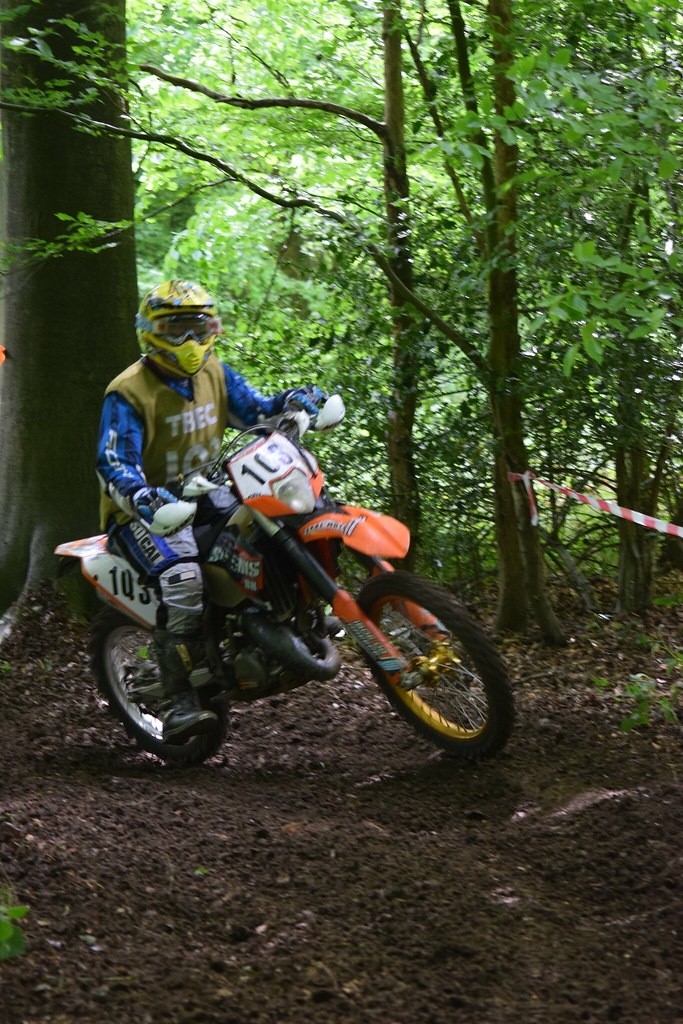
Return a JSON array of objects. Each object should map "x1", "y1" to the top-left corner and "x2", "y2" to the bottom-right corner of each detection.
[
  {"x1": 274, "y1": 385, "x2": 326, "y2": 414},
  {"x1": 129, "y1": 484, "x2": 178, "y2": 525}
]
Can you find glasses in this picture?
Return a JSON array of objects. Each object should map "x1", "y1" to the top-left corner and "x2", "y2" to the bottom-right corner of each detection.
[{"x1": 139, "y1": 312, "x2": 213, "y2": 345}]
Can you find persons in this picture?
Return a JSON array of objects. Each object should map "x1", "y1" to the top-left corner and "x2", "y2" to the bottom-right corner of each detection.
[{"x1": 94, "y1": 279, "x2": 329, "y2": 744}]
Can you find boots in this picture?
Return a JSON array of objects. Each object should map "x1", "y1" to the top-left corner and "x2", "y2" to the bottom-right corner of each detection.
[{"x1": 153, "y1": 627, "x2": 218, "y2": 744}]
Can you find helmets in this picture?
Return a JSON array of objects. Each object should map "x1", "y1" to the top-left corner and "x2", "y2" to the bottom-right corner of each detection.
[{"x1": 135, "y1": 279, "x2": 223, "y2": 377}]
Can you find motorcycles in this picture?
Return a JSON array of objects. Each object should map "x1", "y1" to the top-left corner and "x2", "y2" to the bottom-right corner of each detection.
[{"x1": 53, "y1": 392, "x2": 518, "y2": 772}]
